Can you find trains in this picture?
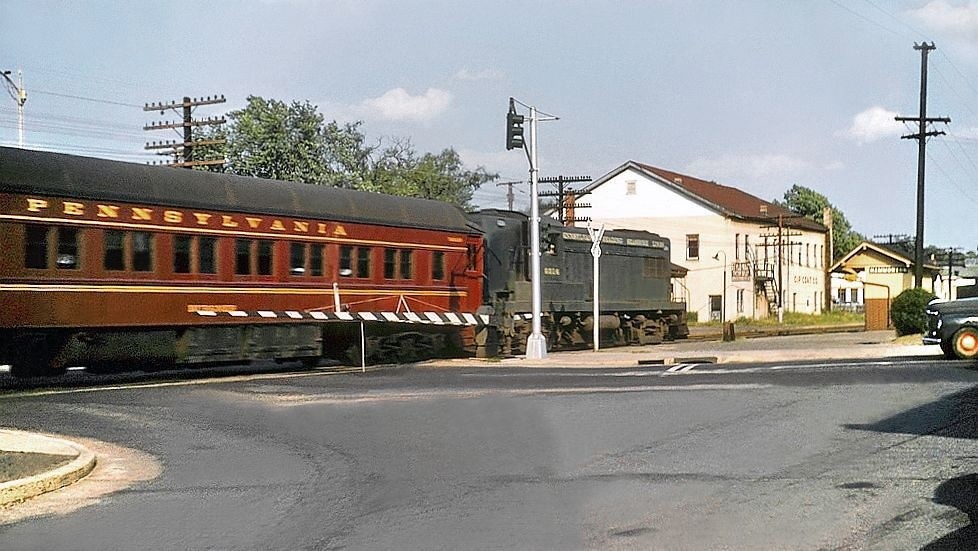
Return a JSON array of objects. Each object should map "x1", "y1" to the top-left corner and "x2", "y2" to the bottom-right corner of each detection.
[{"x1": 0, "y1": 146, "x2": 690, "y2": 380}]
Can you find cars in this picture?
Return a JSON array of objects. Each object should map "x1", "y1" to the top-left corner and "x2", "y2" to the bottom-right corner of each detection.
[{"x1": 921, "y1": 295, "x2": 978, "y2": 361}]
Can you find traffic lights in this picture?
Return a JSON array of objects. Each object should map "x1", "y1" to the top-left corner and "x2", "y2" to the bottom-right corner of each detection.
[{"x1": 506, "y1": 111, "x2": 524, "y2": 151}]
